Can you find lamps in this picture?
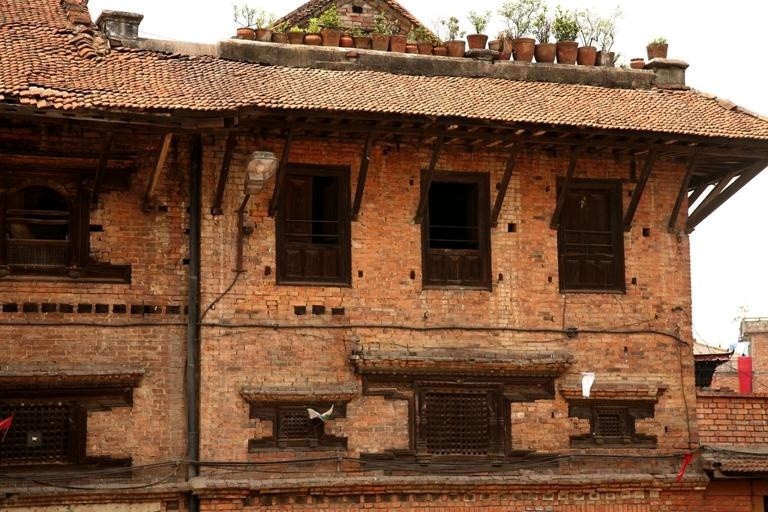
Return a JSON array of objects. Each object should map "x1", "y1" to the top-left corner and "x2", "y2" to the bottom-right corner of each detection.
[{"x1": 231, "y1": 150, "x2": 279, "y2": 273}]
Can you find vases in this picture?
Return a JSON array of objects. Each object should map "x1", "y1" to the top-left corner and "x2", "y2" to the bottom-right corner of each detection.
[{"x1": 630, "y1": 58, "x2": 645, "y2": 69}]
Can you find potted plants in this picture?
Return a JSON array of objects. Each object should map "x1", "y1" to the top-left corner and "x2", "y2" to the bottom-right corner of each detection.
[{"x1": 646, "y1": 36, "x2": 668, "y2": 60}]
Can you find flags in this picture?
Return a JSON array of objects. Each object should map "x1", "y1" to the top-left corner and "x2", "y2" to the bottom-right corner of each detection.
[
  {"x1": 0, "y1": 414, "x2": 15, "y2": 431},
  {"x1": 307, "y1": 406, "x2": 334, "y2": 423},
  {"x1": 580, "y1": 371, "x2": 595, "y2": 398},
  {"x1": 676, "y1": 455, "x2": 691, "y2": 482}
]
